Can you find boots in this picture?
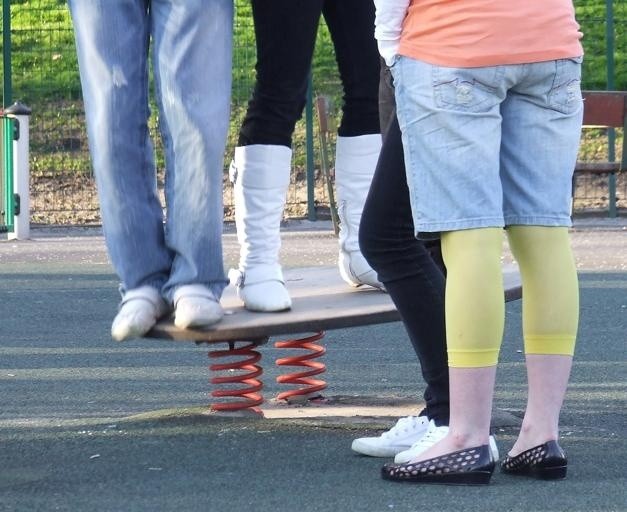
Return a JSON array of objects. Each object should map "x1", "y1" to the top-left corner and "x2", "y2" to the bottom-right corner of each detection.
[
  {"x1": 228, "y1": 144, "x2": 293, "y2": 311},
  {"x1": 335, "y1": 133, "x2": 389, "y2": 293}
]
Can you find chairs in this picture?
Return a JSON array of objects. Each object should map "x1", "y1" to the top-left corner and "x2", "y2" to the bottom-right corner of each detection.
[{"x1": 316, "y1": 96, "x2": 338, "y2": 235}]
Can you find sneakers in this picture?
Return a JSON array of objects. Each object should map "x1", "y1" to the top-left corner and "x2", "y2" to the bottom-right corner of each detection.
[
  {"x1": 173, "y1": 284, "x2": 223, "y2": 329},
  {"x1": 111, "y1": 285, "x2": 167, "y2": 341},
  {"x1": 351, "y1": 415, "x2": 568, "y2": 485}
]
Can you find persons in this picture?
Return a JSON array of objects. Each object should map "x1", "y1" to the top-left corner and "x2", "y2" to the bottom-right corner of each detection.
[
  {"x1": 370, "y1": 0, "x2": 586, "y2": 485},
  {"x1": 63, "y1": 0, "x2": 238, "y2": 343},
  {"x1": 350, "y1": 0, "x2": 503, "y2": 464},
  {"x1": 232, "y1": 0, "x2": 398, "y2": 317}
]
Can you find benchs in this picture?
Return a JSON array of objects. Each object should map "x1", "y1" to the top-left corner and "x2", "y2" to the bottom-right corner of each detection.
[{"x1": 574, "y1": 89, "x2": 627, "y2": 218}]
